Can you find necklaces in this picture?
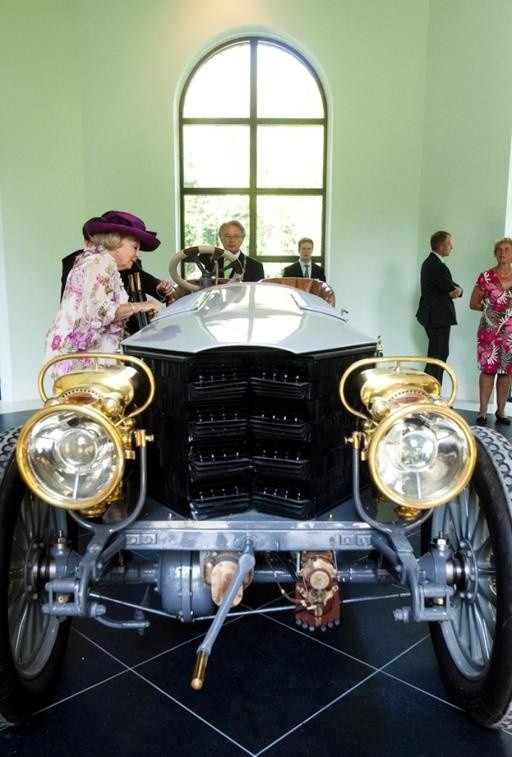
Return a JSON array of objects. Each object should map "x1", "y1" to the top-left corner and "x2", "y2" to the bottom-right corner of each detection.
[{"x1": 496, "y1": 266, "x2": 512, "y2": 278}]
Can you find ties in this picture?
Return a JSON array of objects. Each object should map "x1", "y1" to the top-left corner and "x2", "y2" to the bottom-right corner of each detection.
[{"x1": 304, "y1": 265, "x2": 310, "y2": 278}]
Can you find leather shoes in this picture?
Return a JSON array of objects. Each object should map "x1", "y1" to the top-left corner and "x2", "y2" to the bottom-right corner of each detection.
[
  {"x1": 477, "y1": 413, "x2": 487, "y2": 425},
  {"x1": 496, "y1": 411, "x2": 510, "y2": 424}
]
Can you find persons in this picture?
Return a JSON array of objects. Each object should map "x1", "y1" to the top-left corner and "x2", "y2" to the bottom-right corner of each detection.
[
  {"x1": 40, "y1": 210, "x2": 160, "y2": 384},
  {"x1": 416, "y1": 231, "x2": 463, "y2": 385},
  {"x1": 60, "y1": 249, "x2": 174, "y2": 336},
  {"x1": 470, "y1": 237, "x2": 512, "y2": 427},
  {"x1": 205, "y1": 220, "x2": 264, "y2": 282},
  {"x1": 283, "y1": 237, "x2": 326, "y2": 282}
]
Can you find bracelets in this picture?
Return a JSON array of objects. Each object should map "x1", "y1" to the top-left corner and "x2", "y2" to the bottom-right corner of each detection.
[
  {"x1": 130, "y1": 302, "x2": 135, "y2": 314},
  {"x1": 481, "y1": 303, "x2": 484, "y2": 311}
]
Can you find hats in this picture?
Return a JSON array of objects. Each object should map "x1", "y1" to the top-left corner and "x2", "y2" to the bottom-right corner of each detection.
[{"x1": 85, "y1": 211, "x2": 160, "y2": 251}]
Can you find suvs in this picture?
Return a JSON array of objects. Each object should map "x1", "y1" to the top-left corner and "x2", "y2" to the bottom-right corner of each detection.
[{"x1": 0, "y1": 239, "x2": 512, "y2": 731}]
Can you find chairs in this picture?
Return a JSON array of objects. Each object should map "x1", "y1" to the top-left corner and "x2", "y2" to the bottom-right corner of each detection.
[{"x1": 166, "y1": 274, "x2": 336, "y2": 310}]
